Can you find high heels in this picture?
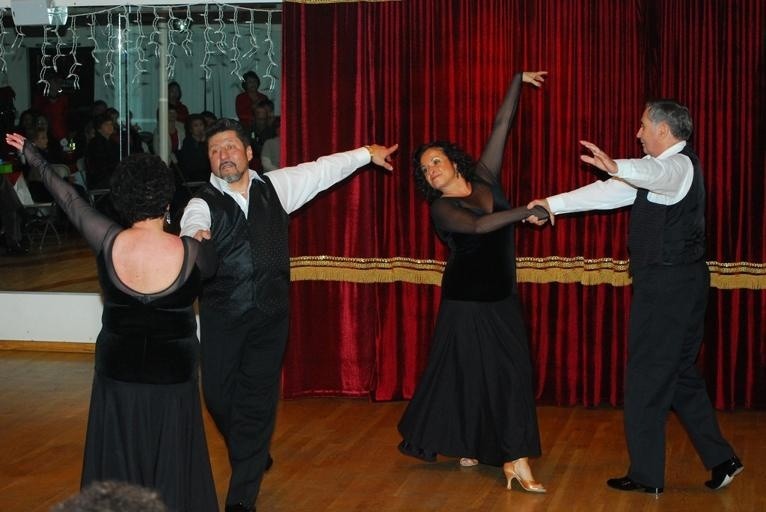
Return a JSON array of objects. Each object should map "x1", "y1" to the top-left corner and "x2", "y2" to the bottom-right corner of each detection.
[{"x1": 502, "y1": 459, "x2": 548, "y2": 495}]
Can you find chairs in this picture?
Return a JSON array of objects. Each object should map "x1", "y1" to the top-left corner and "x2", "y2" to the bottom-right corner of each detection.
[{"x1": 0, "y1": 122, "x2": 209, "y2": 258}]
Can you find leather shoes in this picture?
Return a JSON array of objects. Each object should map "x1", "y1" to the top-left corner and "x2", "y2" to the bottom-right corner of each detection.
[
  {"x1": 6, "y1": 245, "x2": 30, "y2": 256},
  {"x1": 705, "y1": 458, "x2": 744, "y2": 491},
  {"x1": 607, "y1": 475, "x2": 664, "y2": 495},
  {"x1": 21, "y1": 214, "x2": 51, "y2": 233}
]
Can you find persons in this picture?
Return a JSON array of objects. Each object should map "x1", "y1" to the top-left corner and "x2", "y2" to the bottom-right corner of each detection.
[
  {"x1": 520, "y1": 99, "x2": 746, "y2": 494},
  {"x1": 179, "y1": 117, "x2": 400, "y2": 512},
  {"x1": 4, "y1": 130, "x2": 220, "y2": 512},
  {"x1": 397, "y1": 69, "x2": 550, "y2": 494}
]
